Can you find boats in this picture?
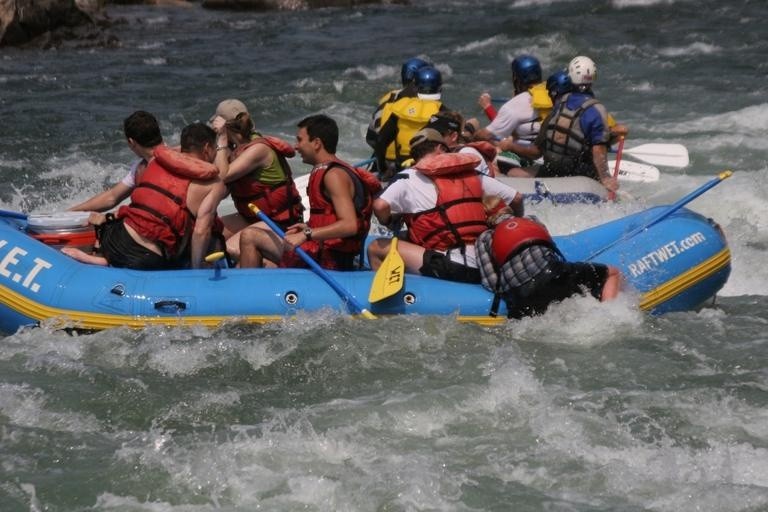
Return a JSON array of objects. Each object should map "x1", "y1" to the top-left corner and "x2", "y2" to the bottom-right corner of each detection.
[
  {"x1": 1, "y1": 203, "x2": 730, "y2": 334},
  {"x1": 365, "y1": 171, "x2": 612, "y2": 204}
]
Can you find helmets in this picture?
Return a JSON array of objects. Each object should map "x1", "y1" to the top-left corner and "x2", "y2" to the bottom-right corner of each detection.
[
  {"x1": 511, "y1": 54, "x2": 541, "y2": 83},
  {"x1": 401, "y1": 57, "x2": 429, "y2": 80},
  {"x1": 546, "y1": 69, "x2": 572, "y2": 104},
  {"x1": 414, "y1": 65, "x2": 443, "y2": 87},
  {"x1": 568, "y1": 55, "x2": 598, "y2": 85}
]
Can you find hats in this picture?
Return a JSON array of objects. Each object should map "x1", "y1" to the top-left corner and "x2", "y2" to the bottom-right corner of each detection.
[
  {"x1": 208, "y1": 98, "x2": 249, "y2": 124},
  {"x1": 407, "y1": 128, "x2": 451, "y2": 154}
]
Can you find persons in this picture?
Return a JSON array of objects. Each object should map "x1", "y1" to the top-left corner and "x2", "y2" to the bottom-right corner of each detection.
[
  {"x1": 365, "y1": 53, "x2": 633, "y2": 192},
  {"x1": 472, "y1": 195, "x2": 621, "y2": 317}
]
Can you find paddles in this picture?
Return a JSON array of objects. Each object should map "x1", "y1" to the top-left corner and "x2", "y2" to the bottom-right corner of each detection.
[
  {"x1": 294, "y1": 157, "x2": 377, "y2": 199},
  {"x1": 492, "y1": 94, "x2": 690, "y2": 183},
  {"x1": 368, "y1": 217, "x2": 404, "y2": 303}
]
